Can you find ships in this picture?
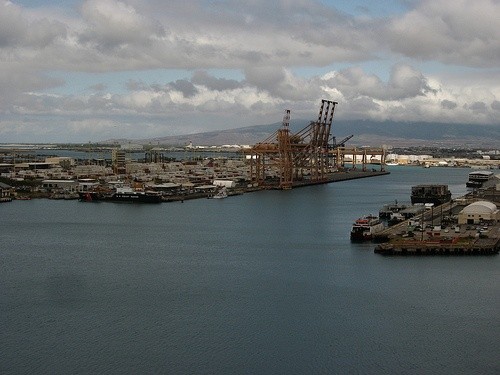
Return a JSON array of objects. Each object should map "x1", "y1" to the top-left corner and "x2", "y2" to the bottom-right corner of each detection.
[{"x1": 76, "y1": 182, "x2": 162, "y2": 203}]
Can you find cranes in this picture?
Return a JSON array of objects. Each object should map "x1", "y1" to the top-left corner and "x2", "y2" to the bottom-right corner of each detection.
[{"x1": 251, "y1": 96, "x2": 354, "y2": 190}]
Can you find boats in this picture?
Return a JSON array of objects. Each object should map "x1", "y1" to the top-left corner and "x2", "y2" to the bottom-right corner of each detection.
[
  {"x1": 410, "y1": 185, "x2": 451, "y2": 206},
  {"x1": 464, "y1": 171, "x2": 494, "y2": 190},
  {"x1": 350, "y1": 215, "x2": 384, "y2": 243},
  {"x1": 380, "y1": 203, "x2": 397, "y2": 218},
  {"x1": 386, "y1": 205, "x2": 425, "y2": 227},
  {"x1": 212, "y1": 192, "x2": 228, "y2": 199}
]
